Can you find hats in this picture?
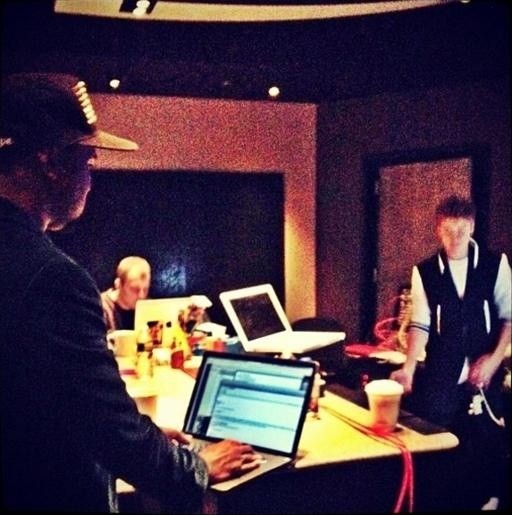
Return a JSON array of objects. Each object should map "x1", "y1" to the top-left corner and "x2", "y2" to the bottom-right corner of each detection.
[{"x1": 1, "y1": 72, "x2": 138, "y2": 151}]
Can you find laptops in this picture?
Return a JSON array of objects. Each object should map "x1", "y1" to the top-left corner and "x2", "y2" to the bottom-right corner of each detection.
[
  {"x1": 133, "y1": 297, "x2": 193, "y2": 343},
  {"x1": 180, "y1": 351, "x2": 316, "y2": 493},
  {"x1": 219, "y1": 283, "x2": 346, "y2": 354}
]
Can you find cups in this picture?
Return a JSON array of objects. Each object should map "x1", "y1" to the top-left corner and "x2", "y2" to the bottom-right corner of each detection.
[
  {"x1": 106, "y1": 329, "x2": 139, "y2": 358},
  {"x1": 364, "y1": 378, "x2": 404, "y2": 433}
]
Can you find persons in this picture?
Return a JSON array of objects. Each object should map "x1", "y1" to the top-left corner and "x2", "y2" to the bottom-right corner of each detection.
[
  {"x1": 387, "y1": 194, "x2": 511, "y2": 513},
  {"x1": 1, "y1": 71, "x2": 264, "y2": 515},
  {"x1": 98, "y1": 255, "x2": 153, "y2": 334}
]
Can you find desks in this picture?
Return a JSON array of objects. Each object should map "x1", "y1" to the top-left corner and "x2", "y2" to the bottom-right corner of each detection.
[{"x1": 107, "y1": 363, "x2": 460, "y2": 513}]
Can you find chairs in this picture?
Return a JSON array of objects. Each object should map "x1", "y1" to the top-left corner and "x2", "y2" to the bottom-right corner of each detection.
[{"x1": 342, "y1": 289, "x2": 428, "y2": 387}]
